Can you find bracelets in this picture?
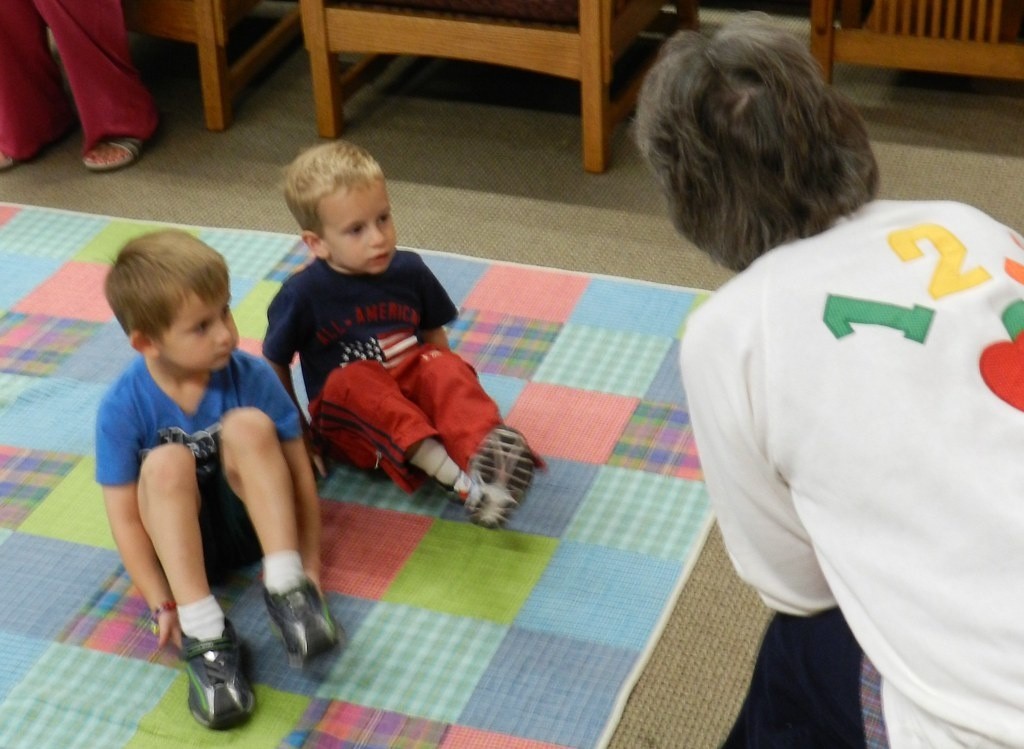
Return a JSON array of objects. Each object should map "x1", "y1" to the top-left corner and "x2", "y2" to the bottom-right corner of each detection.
[{"x1": 147, "y1": 601, "x2": 175, "y2": 625}]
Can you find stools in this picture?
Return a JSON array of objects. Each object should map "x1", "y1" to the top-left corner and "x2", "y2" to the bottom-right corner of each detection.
[{"x1": 119, "y1": 1, "x2": 699, "y2": 174}]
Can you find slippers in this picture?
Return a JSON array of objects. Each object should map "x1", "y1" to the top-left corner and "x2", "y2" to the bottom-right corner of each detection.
[
  {"x1": 83, "y1": 136, "x2": 144, "y2": 171},
  {"x1": 0, "y1": 130, "x2": 63, "y2": 168}
]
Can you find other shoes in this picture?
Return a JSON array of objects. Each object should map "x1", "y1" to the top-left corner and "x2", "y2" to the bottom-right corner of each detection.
[
  {"x1": 464, "y1": 426, "x2": 533, "y2": 529},
  {"x1": 442, "y1": 470, "x2": 470, "y2": 504}
]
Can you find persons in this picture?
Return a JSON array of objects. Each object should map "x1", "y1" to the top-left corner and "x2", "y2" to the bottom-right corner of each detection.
[
  {"x1": 631, "y1": 24, "x2": 1022, "y2": 749},
  {"x1": 0, "y1": 1, "x2": 160, "y2": 176},
  {"x1": 256, "y1": 138, "x2": 538, "y2": 530},
  {"x1": 93, "y1": 229, "x2": 346, "y2": 731}
]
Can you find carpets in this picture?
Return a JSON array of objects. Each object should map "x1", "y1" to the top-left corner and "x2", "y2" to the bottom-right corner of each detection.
[{"x1": 0, "y1": 203, "x2": 717, "y2": 749}]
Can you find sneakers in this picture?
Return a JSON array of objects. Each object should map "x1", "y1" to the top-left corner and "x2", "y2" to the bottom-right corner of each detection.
[
  {"x1": 264, "y1": 576, "x2": 337, "y2": 658},
  {"x1": 179, "y1": 617, "x2": 254, "y2": 727}
]
